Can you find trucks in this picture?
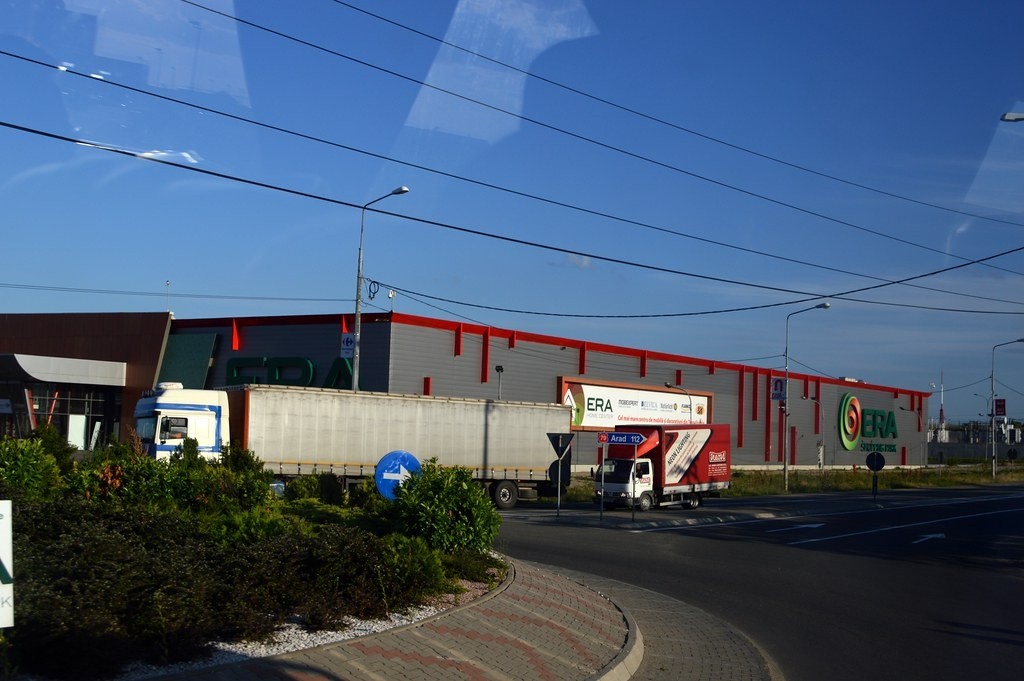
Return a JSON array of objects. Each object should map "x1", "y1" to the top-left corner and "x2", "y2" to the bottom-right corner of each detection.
[{"x1": 590, "y1": 422, "x2": 731, "y2": 512}]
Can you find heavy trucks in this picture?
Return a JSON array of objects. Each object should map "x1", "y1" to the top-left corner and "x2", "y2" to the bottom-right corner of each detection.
[{"x1": 134, "y1": 381, "x2": 573, "y2": 511}]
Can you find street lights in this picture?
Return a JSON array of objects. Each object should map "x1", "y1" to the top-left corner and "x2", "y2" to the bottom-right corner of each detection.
[
  {"x1": 350, "y1": 186, "x2": 411, "y2": 390},
  {"x1": 975, "y1": 393, "x2": 998, "y2": 463},
  {"x1": 665, "y1": 381, "x2": 693, "y2": 425},
  {"x1": 782, "y1": 302, "x2": 831, "y2": 492},
  {"x1": 801, "y1": 395, "x2": 826, "y2": 465},
  {"x1": 991, "y1": 338, "x2": 1024, "y2": 480}
]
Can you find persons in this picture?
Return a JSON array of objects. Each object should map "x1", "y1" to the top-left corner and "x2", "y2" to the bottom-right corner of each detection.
[
  {"x1": 166, "y1": 420, "x2": 183, "y2": 438},
  {"x1": 635, "y1": 464, "x2": 646, "y2": 478}
]
